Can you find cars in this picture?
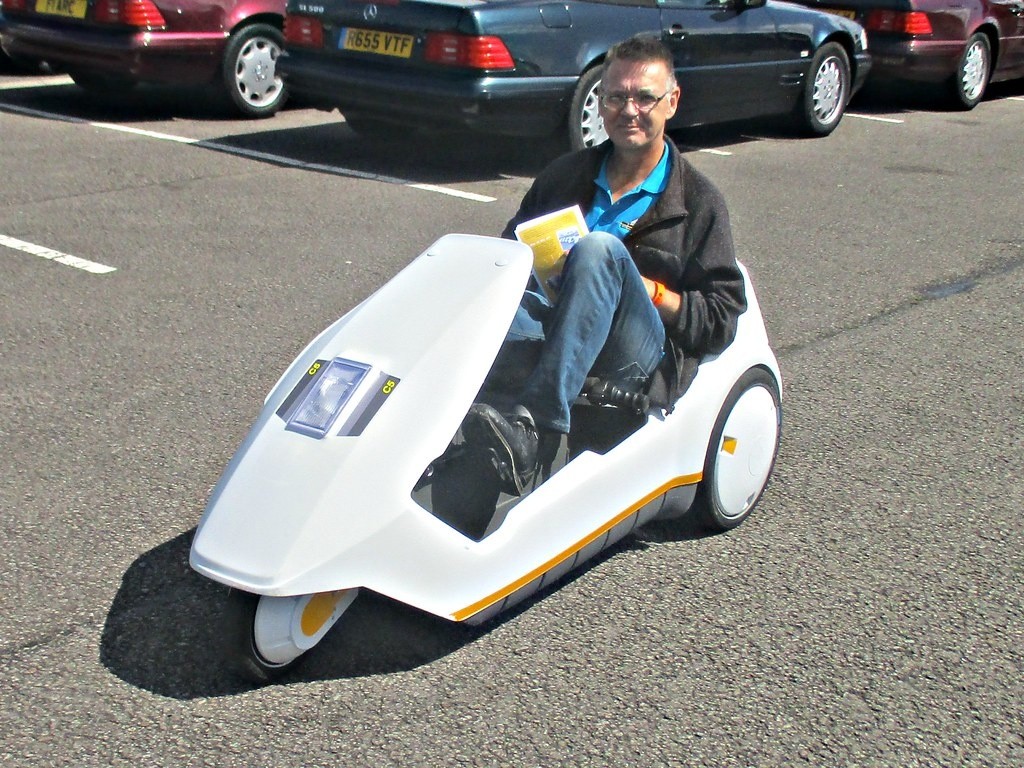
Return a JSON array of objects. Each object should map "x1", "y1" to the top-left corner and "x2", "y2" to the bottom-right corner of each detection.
[
  {"x1": 284, "y1": 1, "x2": 874, "y2": 163},
  {"x1": 0, "y1": 1, "x2": 299, "y2": 115},
  {"x1": 778, "y1": 1, "x2": 1024, "y2": 108}
]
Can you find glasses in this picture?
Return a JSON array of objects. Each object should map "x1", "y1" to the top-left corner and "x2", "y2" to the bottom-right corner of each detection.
[{"x1": 599, "y1": 89, "x2": 673, "y2": 111}]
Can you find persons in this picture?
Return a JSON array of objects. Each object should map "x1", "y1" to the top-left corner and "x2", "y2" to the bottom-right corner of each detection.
[{"x1": 354, "y1": 37, "x2": 747, "y2": 497}]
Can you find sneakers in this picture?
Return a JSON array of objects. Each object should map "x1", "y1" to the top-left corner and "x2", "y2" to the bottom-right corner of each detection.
[{"x1": 462, "y1": 401, "x2": 542, "y2": 497}]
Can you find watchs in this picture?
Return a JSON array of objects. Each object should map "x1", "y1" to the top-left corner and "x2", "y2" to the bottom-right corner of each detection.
[{"x1": 642, "y1": 279, "x2": 668, "y2": 311}]
